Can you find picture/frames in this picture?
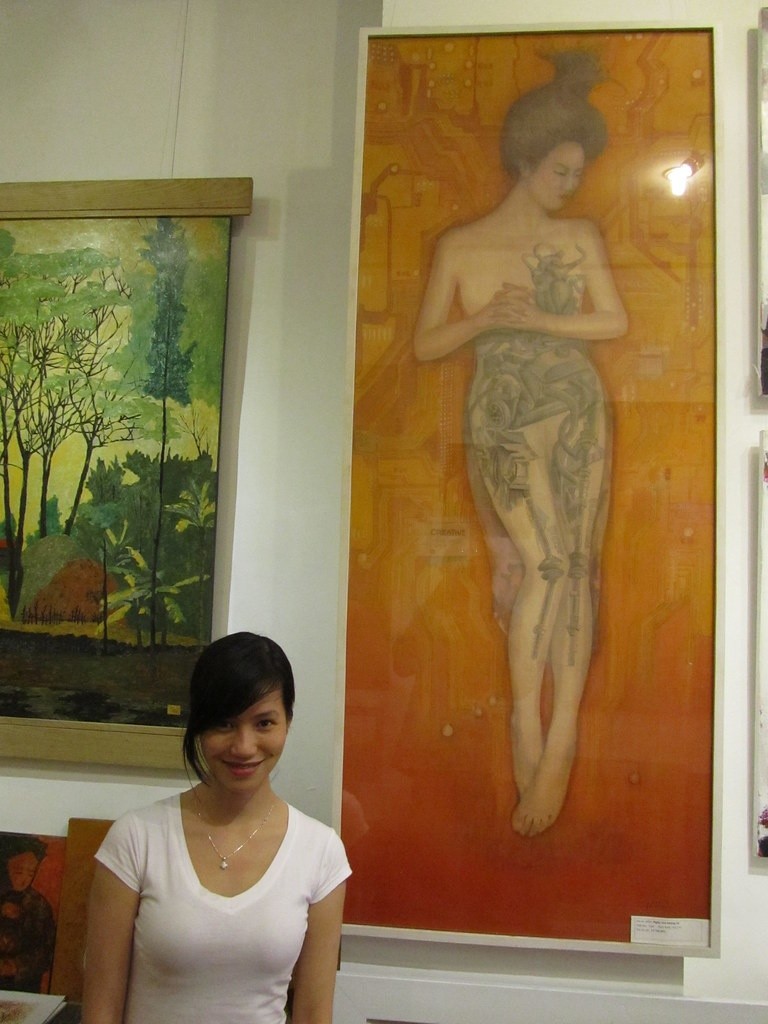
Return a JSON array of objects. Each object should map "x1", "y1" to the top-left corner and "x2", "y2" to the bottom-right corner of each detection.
[
  {"x1": 334, "y1": 24, "x2": 723, "y2": 960},
  {"x1": 0, "y1": 174, "x2": 253, "y2": 770}
]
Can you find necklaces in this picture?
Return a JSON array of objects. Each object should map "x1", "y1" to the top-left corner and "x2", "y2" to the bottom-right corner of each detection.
[{"x1": 196, "y1": 782, "x2": 277, "y2": 870}]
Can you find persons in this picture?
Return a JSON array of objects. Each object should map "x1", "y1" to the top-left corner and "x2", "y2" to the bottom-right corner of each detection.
[{"x1": 82, "y1": 631, "x2": 353, "y2": 1024}]
